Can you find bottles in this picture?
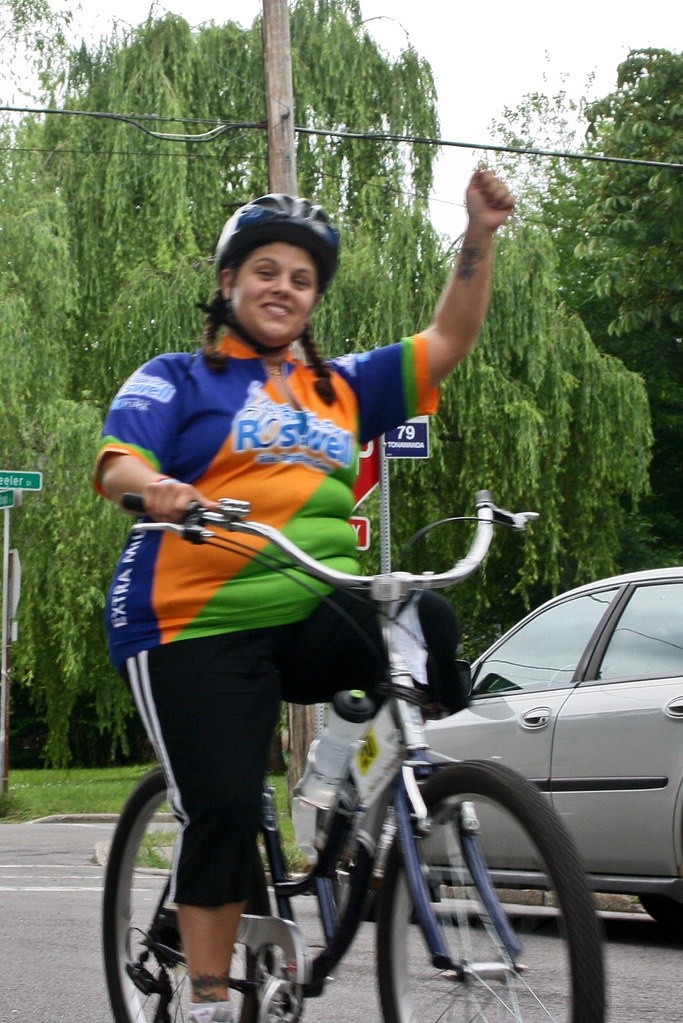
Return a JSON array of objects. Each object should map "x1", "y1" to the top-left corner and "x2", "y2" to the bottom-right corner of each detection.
[{"x1": 298, "y1": 687, "x2": 373, "y2": 810}]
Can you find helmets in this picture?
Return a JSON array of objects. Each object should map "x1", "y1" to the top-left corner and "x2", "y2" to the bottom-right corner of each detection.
[{"x1": 210, "y1": 183, "x2": 339, "y2": 284}]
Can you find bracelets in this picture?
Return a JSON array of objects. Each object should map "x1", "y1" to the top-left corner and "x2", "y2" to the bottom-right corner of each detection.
[{"x1": 148, "y1": 475, "x2": 173, "y2": 485}]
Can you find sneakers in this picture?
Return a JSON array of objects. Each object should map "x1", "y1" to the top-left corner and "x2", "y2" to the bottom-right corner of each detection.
[{"x1": 294, "y1": 782, "x2": 374, "y2": 865}]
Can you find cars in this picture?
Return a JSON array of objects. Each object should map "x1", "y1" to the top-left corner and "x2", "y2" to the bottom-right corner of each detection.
[{"x1": 344, "y1": 565, "x2": 683, "y2": 944}]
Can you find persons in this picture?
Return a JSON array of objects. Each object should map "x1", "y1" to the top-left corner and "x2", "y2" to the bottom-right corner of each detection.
[{"x1": 91, "y1": 161, "x2": 517, "y2": 1023}]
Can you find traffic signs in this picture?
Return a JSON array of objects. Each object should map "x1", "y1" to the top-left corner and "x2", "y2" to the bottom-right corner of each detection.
[
  {"x1": 0, "y1": 468, "x2": 43, "y2": 492},
  {"x1": 1, "y1": 489, "x2": 24, "y2": 509}
]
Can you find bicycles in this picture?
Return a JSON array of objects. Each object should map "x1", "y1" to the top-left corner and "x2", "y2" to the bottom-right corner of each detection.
[{"x1": 97, "y1": 486, "x2": 607, "y2": 1022}]
